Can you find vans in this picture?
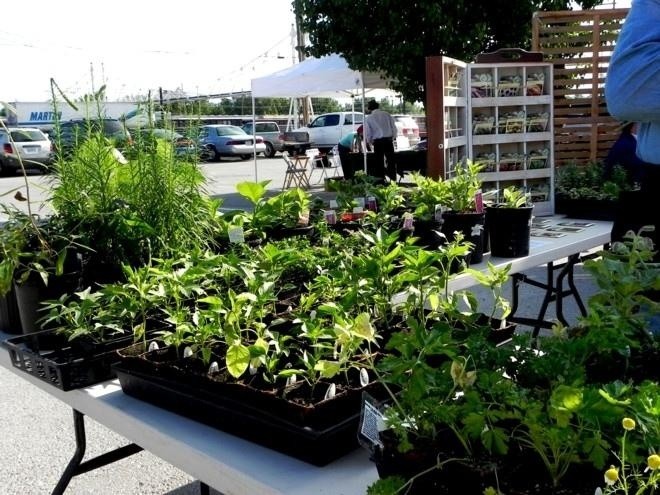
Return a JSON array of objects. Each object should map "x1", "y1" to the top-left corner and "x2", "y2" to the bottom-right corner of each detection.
[{"x1": 55, "y1": 118, "x2": 132, "y2": 161}]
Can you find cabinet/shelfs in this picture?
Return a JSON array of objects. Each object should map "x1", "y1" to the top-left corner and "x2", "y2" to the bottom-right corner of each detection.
[{"x1": 426, "y1": 48, "x2": 556, "y2": 218}]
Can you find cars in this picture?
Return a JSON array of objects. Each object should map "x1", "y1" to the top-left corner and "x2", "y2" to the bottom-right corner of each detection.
[
  {"x1": 245, "y1": 120, "x2": 313, "y2": 159},
  {"x1": 393, "y1": 115, "x2": 432, "y2": 149},
  {"x1": 198, "y1": 126, "x2": 264, "y2": 158},
  {"x1": 134, "y1": 128, "x2": 198, "y2": 163}
]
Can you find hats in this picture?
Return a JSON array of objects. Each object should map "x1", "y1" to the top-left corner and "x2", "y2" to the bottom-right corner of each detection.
[{"x1": 368, "y1": 99, "x2": 379, "y2": 109}]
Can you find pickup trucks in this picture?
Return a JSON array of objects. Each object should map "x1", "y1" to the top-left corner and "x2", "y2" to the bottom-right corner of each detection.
[{"x1": 294, "y1": 112, "x2": 410, "y2": 154}]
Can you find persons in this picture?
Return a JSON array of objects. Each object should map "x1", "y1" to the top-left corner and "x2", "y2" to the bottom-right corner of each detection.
[
  {"x1": 604, "y1": 123, "x2": 641, "y2": 189},
  {"x1": 337, "y1": 125, "x2": 366, "y2": 179},
  {"x1": 605, "y1": 0, "x2": 659, "y2": 264},
  {"x1": 362, "y1": 100, "x2": 398, "y2": 181}
]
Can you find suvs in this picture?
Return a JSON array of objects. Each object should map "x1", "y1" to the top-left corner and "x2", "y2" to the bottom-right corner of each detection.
[{"x1": 0, "y1": 129, "x2": 52, "y2": 172}]
